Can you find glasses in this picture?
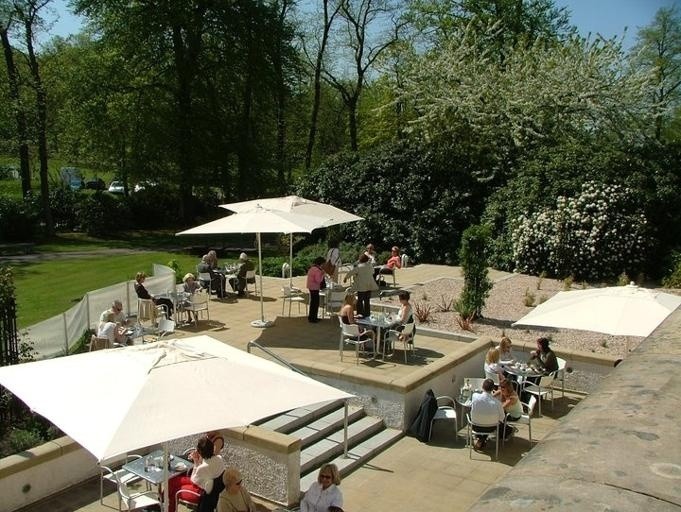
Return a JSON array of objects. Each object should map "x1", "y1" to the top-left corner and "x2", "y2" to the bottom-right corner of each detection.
[
  {"x1": 236, "y1": 479, "x2": 242, "y2": 485},
  {"x1": 320, "y1": 474, "x2": 332, "y2": 480}
]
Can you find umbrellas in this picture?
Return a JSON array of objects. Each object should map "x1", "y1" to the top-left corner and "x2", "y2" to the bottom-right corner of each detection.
[
  {"x1": 1, "y1": 334, "x2": 359, "y2": 462},
  {"x1": 174, "y1": 203, "x2": 333, "y2": 234},
  {"x1": 511, "y1": 281, "x2": 681, "y2": 337},
  {"x1": 218, "y1": 194, "x2": 366, "y2": 224}
]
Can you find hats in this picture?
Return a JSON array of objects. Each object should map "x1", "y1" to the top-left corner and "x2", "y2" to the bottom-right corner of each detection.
[{"x1": 183, "y1": 272, "x2": 195, "y2": 282}]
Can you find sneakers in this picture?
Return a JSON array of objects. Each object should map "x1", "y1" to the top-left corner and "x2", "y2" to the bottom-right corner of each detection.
[
  {"x1": 505, "y1": 427, "x2": 516, "y2": 442},
  {"x1": 474, "y1": 438, "x2": 488, "y2": 450}
]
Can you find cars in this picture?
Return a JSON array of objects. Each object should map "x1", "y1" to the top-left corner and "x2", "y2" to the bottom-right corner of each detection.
[
  {"x1": 2, "y1": 166, "x2": 23, "y2": 180},
  {"x1": 108, "y1": 180, "x2": 127, "y2": 194},
  {"x1": 135, "y1": 181, "x2": 151, "y2": 194},
  {"x1": 85, "y1": 180, "x2": 108, "y2": 190}
]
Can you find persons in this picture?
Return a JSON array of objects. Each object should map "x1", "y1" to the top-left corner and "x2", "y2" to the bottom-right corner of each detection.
[
  {"x1": 97, "y1": 249, "x2": 256, "y2": 340},
  {"x1": 465, "y1": 335, "x2": 558, "y2": 450},
  {"x1": 299, "y1": 460, "x2": 344, "y2": 512},
  {"x1": 189, "y1": 440, "x2": 226, "y2": 512},
  {"x1": 306, "y1": 238, "x2": 416, "y2": 357},
  {"x1": 215, "y1": 468, "x2": 256, "y2": 512}
]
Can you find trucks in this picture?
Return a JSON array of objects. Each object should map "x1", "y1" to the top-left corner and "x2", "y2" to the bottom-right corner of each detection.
[{"x1": 61, "y1": 167, "x2": 86, "y2": 192}]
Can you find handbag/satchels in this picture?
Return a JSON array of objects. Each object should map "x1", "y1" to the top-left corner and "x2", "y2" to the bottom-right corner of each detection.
[{"x1": 319, "y1": 277, "x2": 326, "y2": 290}]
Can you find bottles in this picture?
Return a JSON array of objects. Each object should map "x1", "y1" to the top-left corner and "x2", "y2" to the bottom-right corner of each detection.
[
  {"x1": 146, "y1": 453, "x2": 173, "y2": 471},
  {"x1": 462, "y1": 378, "x2": 481, "y2": 399}
]
